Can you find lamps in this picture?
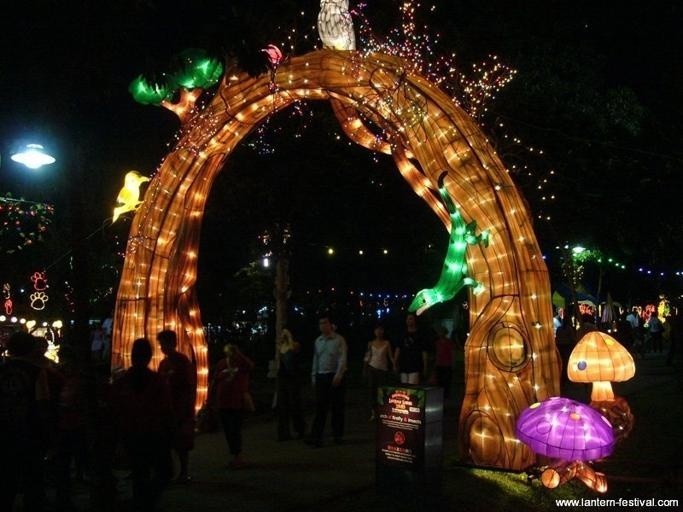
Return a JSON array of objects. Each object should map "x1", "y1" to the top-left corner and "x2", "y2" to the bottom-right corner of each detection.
[{"x1": 8, "y1": 140, "x2": 57, "y2": 172}]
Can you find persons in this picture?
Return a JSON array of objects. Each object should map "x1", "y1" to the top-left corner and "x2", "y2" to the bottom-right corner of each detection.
[
  {"x1": 3, "y1": 311, "x2": 453, "y2": 489},
  {"x1": 551, "y1": 303, "x2": 662, "y2": 354}
]
